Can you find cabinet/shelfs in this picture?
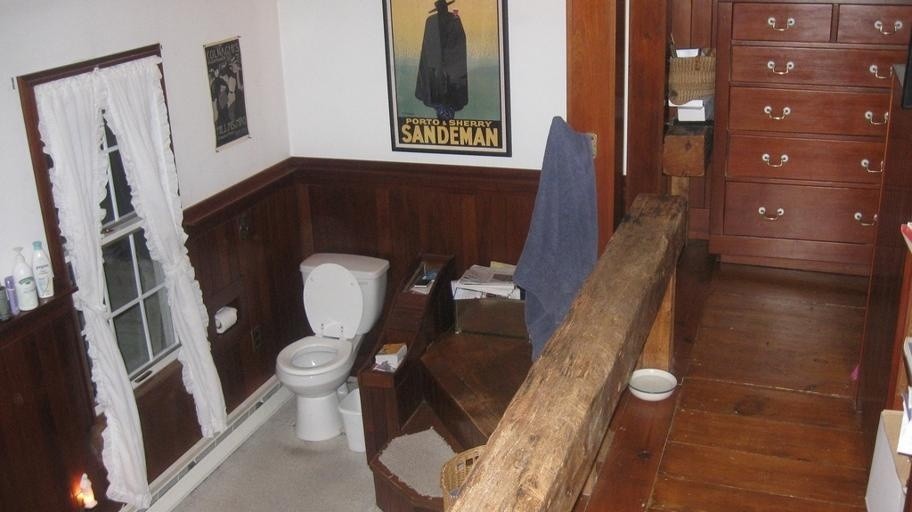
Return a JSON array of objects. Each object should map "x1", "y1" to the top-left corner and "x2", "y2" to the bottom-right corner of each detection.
[
  {"x1": 885, "y1": 224, "x2": 912, "y2": 512},
  {"x1": 707, "y1": 0, "x2": 912, "y2": 278}
]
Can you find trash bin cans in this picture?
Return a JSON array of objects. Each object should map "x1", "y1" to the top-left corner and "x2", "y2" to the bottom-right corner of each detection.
[{"x1": 337, "y1": 388, "x2": 365, "y2": 453}]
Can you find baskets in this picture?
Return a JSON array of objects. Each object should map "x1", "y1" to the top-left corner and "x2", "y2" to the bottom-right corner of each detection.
[
  {"x1": 668, "y1": 51, "x2": 717, "y2": 105},
  {"x1": 440, "y1": 443, "x2": 490, "y2": 511}
]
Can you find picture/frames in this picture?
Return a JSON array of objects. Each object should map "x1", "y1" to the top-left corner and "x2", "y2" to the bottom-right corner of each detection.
[{"x1": 382, "y1": 0, "x2": 512, "y2": 157}]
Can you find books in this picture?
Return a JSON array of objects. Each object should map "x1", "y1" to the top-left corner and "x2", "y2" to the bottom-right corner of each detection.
[{"x1": 445, "y1": 260, "x2": 523, "y2": 305}]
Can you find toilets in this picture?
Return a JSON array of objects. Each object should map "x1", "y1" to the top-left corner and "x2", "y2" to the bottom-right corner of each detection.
[{"x1": 274, "y1": 253, "x2": 391, "y2": 443}]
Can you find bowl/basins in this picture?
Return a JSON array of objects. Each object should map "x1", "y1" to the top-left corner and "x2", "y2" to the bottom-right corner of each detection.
[{"x1": 628, "y1": 367, "x2": 677, "y2": 403}]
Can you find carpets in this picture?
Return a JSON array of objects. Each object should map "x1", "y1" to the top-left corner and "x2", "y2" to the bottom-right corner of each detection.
[{"x1": 377, "y1": 425, "x2": 460, "y2": 499}]
